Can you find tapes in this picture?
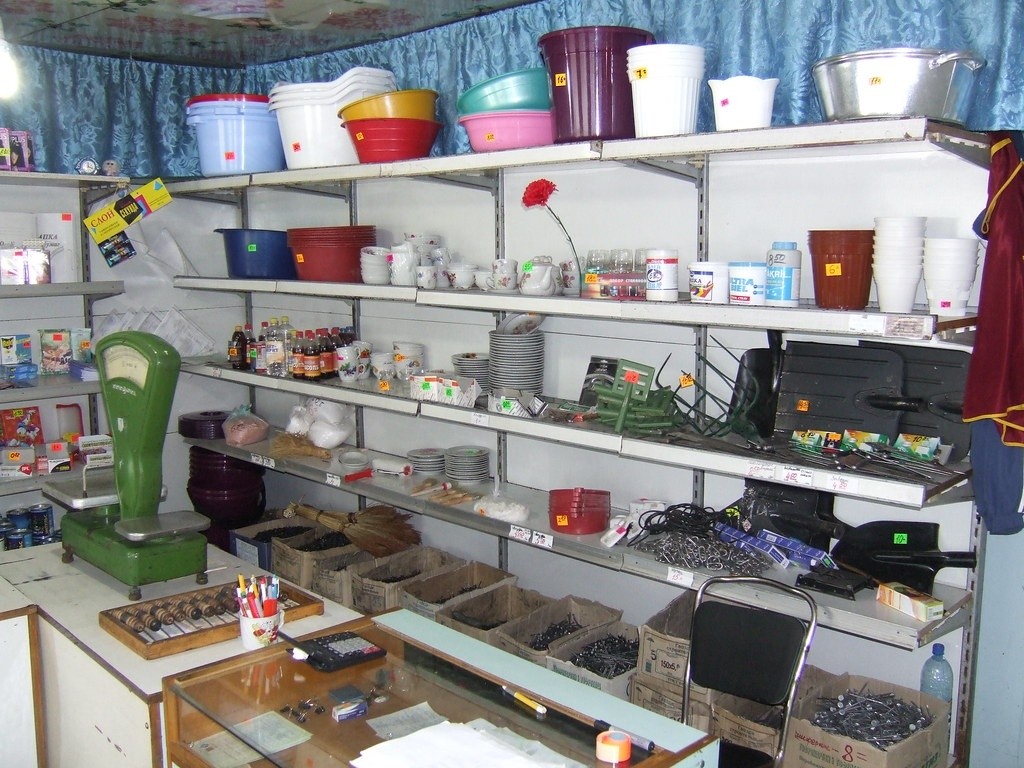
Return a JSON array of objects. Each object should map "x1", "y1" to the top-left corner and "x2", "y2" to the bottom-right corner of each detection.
[{"x1": 595, "y1": 730, "x2": 633, "y2": 763}]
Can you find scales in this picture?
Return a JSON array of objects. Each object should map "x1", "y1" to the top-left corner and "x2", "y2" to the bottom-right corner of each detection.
[{"x1": 60, "y1": 330, "x2": 213, "y2": 603}]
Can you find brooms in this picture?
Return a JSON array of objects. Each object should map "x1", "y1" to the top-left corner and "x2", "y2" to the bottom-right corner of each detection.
[{"x1": 287, "y1": 498, "x2": 423, "y2": 558}]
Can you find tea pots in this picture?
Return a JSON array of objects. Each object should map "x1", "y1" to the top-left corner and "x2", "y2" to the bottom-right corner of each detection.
[{"x1": 519, "y1": 257, "x2": 563, "y2": 297}]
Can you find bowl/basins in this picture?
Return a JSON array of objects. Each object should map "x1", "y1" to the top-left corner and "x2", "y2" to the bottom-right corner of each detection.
[
  {"x1": 371, "y1": 341, "x2": 424, "y2": 382},
  {"x1": 361, "y1": 232, "x2": 493, "y2": 293},
  {"x1": 337, "y1": 90, "x2": 439, "y2": 120},
  {"x1": 337, "y1": 118, "x2": 441, "y2": 165},
  {"x1": 458, "y1": 66, "x2": 556, "y2": 152},
  {"x1": 213, "y1": 225, "x2": 377, "y2": 284},
  {"x1": 338, "y1": 452, "x2": 369, "y2": 471},
  {"x1": 337, "y1": 340, "x2": 372, "y2": 383}
]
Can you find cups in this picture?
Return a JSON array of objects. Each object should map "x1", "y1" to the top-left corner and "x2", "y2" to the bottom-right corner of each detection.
[
  {"x1": 562, "y1": 271, "x2": 581, "y2": 288},
  {"x1": 560, "y1": 256, "x2": 586, "y2": 271},
  {"x1": 871, "y1": 217, "x2": 980, "y2": 316},
  {"x1": 486, "y1": 274, "x2": 517, "y2": 289},
  {"x1": 585, "y1": 247, "x2": 648, "y2": 294},
  {"x1": 491, "y1": 259, "x2": 518, "y2": 273}
]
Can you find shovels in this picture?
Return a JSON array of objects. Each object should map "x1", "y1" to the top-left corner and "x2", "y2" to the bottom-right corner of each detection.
[
  {"x1": 738, "y1": 480, "x2": 852, "y2": 557},
  {"x1": 726, "y1": 329, "x2": 787, "y2": 439},
  {"x1": 858, "y1": 337, "x2": 979, "y2": 465},
  {"x1": 771, "y1": 339, "x2": 926, "y2": 451},
  {"x1": 829, "y1": 520, "x2": 977, "y2": 596}
]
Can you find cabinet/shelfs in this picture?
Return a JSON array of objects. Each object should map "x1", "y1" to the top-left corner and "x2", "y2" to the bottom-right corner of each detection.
[
  {"x1": 0, "y1": 168, "x2": 167, "y2": 544},
  {"x1": 160, "y1": 116, "x2": 994, "y2": 767},
  {"x1": 0, "y1": 536, "x2": 631, "y2": 767}
]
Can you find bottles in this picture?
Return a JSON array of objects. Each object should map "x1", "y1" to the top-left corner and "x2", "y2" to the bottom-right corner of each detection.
[
  {"x1": 330, "y1": 328, "x2": 342, "y2": 377},
  {"x1": 339, "y1": 328, "x2": 345, "y2": 340},
  {"x1": 232, "y1": 317, "x2": 332, "y2": 383},
  {"x1": 343, "y1": 327, "x2": 357, "y2": 346}
]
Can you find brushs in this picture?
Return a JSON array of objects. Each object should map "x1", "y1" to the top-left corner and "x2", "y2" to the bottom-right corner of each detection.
[{"x1": 343, "y1": 454, "x2": 414, "y2": 483}]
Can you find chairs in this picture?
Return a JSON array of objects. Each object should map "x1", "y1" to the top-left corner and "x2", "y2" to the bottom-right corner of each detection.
[{"x1": 681, "y1": 577, "x2": 818, "y2": 766}]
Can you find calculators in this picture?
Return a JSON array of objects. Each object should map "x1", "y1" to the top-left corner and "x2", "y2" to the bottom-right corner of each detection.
[{"x1": 289, "y1": 630, "x2": 387, "y2": 672}]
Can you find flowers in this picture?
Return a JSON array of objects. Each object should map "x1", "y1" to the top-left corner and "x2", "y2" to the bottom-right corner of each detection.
[{"x1": 523, "y1": 178, "x2": 584, "y2": 298}]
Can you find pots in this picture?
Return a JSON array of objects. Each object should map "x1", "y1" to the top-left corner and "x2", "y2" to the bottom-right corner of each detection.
[{"x1": 812, "y1": 48, "x2": 989, "y2": 129}]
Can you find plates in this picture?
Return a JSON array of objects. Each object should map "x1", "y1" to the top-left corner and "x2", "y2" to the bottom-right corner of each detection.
[
  {"x1": 408, "y1": 446, "x2": 490, "y2": 487},
  {"x1": 486, "y1": 288, "x2": 520, "y2": 294},
  {"x1": 450, "y1": 312, "x2": 545, "y2": 396},
  {"x1": 561, "y1": 288, "x2": 581, "y2": 295}
]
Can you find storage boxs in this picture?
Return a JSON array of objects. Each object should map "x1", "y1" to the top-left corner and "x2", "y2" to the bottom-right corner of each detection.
[
  {"x1": 0, "y1": 127, "x2": 36, "y2": 172},
  {"x1": 0, "y1": 328, "x2": 92, "y2": 385},
  {"x1": 1, "y1": 406, "x2": 115, "y2": 479},
  {"x1": 0, "y1": 242, "x2": 52, "y2": 285},
  {"x1": 230, "y1": 517, "x2": 947, "y2": 767}
]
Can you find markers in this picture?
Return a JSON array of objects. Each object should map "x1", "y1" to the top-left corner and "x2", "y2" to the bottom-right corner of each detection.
[{"x1": 594, "y1": 719, "x2": 655, "y2": 752}]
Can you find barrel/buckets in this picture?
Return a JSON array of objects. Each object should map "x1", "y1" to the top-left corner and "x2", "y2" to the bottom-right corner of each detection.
[
  {"x1": 239, "y1": 610, "x2": 284, "y2": 648},
  {"x1": 628, "y1": 44, "x2": 707, "y2": 138},
  {"x1": 537, "y1": 25, "x2": 655, "y2": 144},
  {"x1": 186, "y1": 66, "x2": 395, "y2": 176},
  {"x1": 808, "y1": 229, "x2": 875, "y2": 309},
  {"x1": 709, "y1": 76, "x2": 779, "y2": 133}
]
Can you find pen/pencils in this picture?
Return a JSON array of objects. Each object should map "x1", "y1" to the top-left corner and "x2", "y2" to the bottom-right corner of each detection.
[
  {"x1": 233, "y1": 572, "x2": 280, "y2": 618},
  {"x1": 499, "y1": 684, "x2": 547, "y2": 715}
]
